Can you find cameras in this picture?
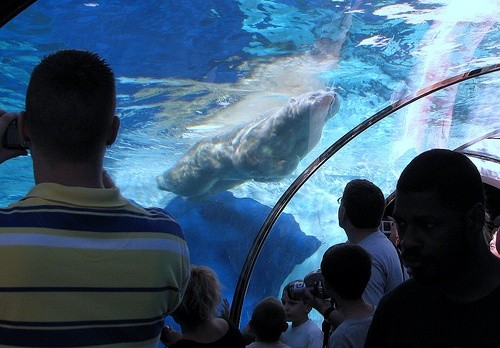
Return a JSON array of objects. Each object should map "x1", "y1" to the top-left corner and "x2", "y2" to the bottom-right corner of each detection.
[
  {"x1": 4, "y1": 119, "x2": 27, "y2": 150},
  {"x1": 381, "y1": 221, "x2": 393, "y2": 233},
  {"x1": 294, "y1": 279, "x2": 330, "y2": 300}
]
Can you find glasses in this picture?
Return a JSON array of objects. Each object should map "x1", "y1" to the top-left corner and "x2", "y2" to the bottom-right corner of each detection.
[{"x1": 337, "y1": 197, "x2": 348, "y2": 216}]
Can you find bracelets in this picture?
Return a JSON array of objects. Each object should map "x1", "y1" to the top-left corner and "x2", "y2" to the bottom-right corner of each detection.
[{"x1": 324, "y1": 306, "x2": 336, "y2": 319}]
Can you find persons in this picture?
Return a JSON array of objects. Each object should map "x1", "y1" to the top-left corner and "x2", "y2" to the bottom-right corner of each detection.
[
  {"x1": 280, "y1": 280, "x2": 325, "y2": 348},
  {"x1": 244, "y1": 297, "x2": 292, "y2": 348},
  {"x1": 321, "y1": 243, "x2": 377, "y2": 348},
  {"x1": 363, "y1": 149, "x2": 500, "y2": 348},
  {"x1": 290, "y1": 178, "x2": 413, "y2": 327},
  {"x1": 0, "y1": 49, "x2": 192, "y2": 348},
  {"x1": 161, "y1": 266, "x2": 244, "y2": 348}
]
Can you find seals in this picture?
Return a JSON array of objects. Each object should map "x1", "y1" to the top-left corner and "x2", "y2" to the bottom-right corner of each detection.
[{"x1": 155, "y1": 91, "x2": 342, "y2": 200}]
